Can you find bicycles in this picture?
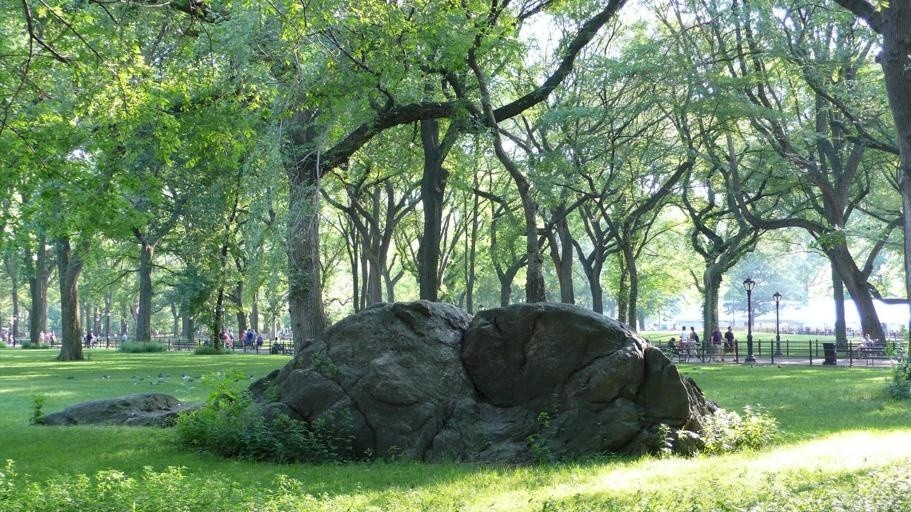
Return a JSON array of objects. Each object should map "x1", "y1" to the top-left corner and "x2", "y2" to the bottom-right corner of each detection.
[
  {"x1": 675, "y1": 343, "x2": 710, "y2": 364},
  {"x1": 657, "y1": 346, "x2": 680, "y2": 361}
]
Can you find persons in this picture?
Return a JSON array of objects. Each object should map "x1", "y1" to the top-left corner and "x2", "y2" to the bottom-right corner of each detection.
[
  {"x1": 83, "y1": 331, "x2": 128, "y2": 349},
  {"x1": 40, "y1": 330, "x2": 57, "y2": 349},
  {"x1": 2, "y1": 331, "x2": 14, "y2": 344},
  {"x1": 222, "y1": 328, "x2": 278, "y2": 351},
  {"x1": 859, "y1": 334, "x2": 875, "y2": 352},
  {"x1": 668, "y1": 326, "x2": 736, "y2": 363}
]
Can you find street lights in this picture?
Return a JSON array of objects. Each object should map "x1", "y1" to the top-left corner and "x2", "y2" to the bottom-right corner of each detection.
[
  {"x1": 771, "y1": 291, "x2": 784, "y2": 359},
  {"x1": 95, "y1": 316, "x2": 99, "y2": 336},
  {"x1": 187, "y1": 314, "x2": 192, "y2": 328},
  {"x1": 742, "y1": 275, "x2": 755, "y2": 359},
  {"x1": 221, "y1": 306, "x2": 225, "y2": 334},
  {"x1": 105, "y1": 310, "x2": 110, "y2": 348},
  {"x1": 11, "y1": 314, "x2": 17, "y2": 346}
]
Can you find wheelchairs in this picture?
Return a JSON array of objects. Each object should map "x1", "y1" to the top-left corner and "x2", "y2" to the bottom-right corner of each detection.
[{"x1": 888, "y1": 341, "x2": 910, "y2": 366}]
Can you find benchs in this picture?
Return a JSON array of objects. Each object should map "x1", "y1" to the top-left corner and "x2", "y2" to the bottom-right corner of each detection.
[
  {"x1": 854, "y1": 350, "x2": 884, "y2": 357},
  {"x1": 272, "y1": 349, "x2": 294, "y2": 355},
  {"x1": 862, "y1": 356, "x2": 900, "y2": 365}
]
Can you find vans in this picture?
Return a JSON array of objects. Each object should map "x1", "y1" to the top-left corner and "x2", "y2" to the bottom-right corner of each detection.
[{"x1": 860, "y1": 322, "x2": 888, "y2": 338}]
